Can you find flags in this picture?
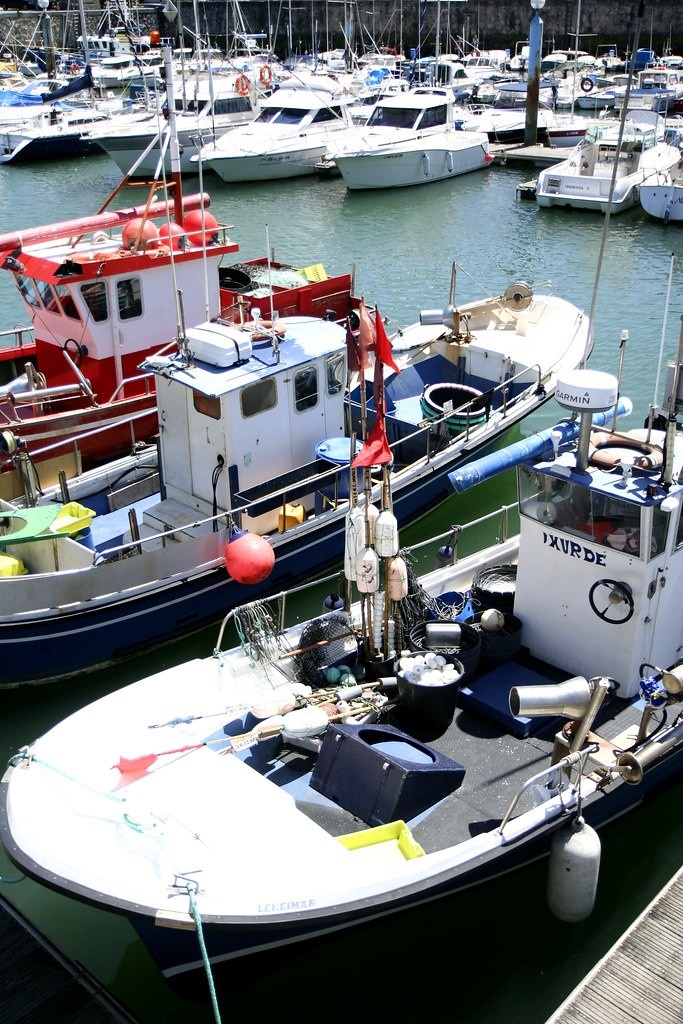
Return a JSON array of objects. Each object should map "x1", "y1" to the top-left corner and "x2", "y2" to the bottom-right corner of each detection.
[{"x1": 346, "y1": 302, "x2": 401, "y2": 375}]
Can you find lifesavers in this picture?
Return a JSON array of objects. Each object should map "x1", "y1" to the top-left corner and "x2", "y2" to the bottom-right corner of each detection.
[
  {"x1": 259, "y1": 65, "x2": 272, "y2": 85},
  {"x1": 580, "y1": 78, "x2": 594, "y2": 92},
  {"x1": 114, "y1": 244, "x2": 170, "y2": 258},
  {"x1": 231, "y1": 321, "x2": 287, "y2": 341},
  {"x1": 584, "y1": 433, "x2": 664, "y2": 470},
  {"x1": 70, "y1": 63, "x2": 80, "y2": 75},
  {"x1": 653, "y1": 66, "x2": 666, "y2": 70},
  {"x1": 66, "y1": 251, "x2": 121, "y2": 268},
  {"x1": 235, "y1": 74, "x2": 250, "y2": 96}
]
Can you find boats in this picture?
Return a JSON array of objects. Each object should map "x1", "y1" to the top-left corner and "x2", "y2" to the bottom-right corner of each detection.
[
  {"x1": 0, "y1": 0, "x2": 683, "y2": 721},
  {"x1": 0, "y1": 0, "x2": 683, "y2": 992}
]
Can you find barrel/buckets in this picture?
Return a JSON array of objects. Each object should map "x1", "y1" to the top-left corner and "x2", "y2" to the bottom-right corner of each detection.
[
  {"x1": 315, "y1": 437, "x2": 366, "y2": 514},
  {"x1": 419, "y1": 382, "x2": 492, "y2": 434},
  {"x1": 408, "y1": 564, "x2": 524, "y2": 684},
  {"x1": 393, "y1": 651, "x2": 464, "y2": 740}
]
